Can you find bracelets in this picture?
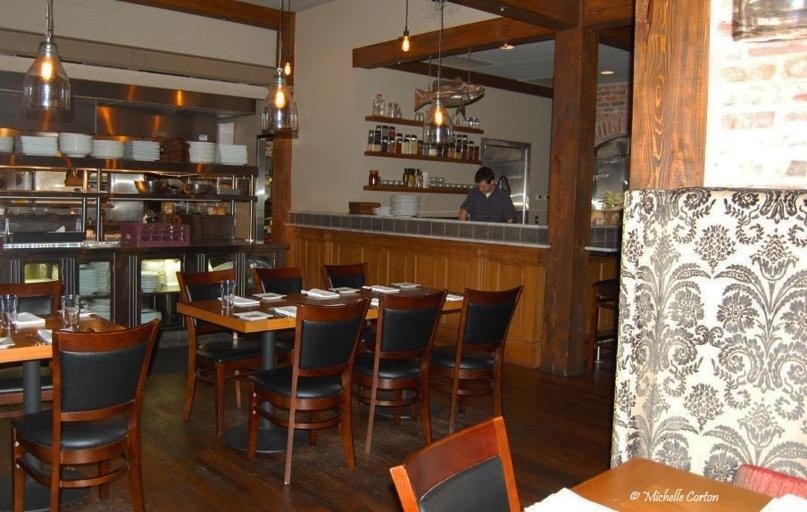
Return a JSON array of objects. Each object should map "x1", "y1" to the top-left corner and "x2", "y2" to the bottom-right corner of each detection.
[
  {"x1": 169, "y1": 189, "x2": 184, "y2": 197},
  {"x1": 141, "y1": 273, "x2": 160, "y2": 292},
  {"x1": 185, "y1": 184, "x2": 212, "y2": 194},
  {"x1": 135, "y1": 181, "x2": 169, "y2": 194}
]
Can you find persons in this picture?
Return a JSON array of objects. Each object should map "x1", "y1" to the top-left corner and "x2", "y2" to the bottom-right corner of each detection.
[{"x1": 459, "y1": 167, "x2": 517, "y2": 223}]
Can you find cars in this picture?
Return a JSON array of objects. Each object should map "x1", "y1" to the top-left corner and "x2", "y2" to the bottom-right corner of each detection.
[
  {"x1": 61, "y1": 294, "x2": 80, "y2": 330},
  {"x1": 177, "y1": 224, "x2": 184, "y2": 240},
  {"x1": 220, "y1": 280, "x2": 236, "y2": 309},
  {"x1": 0, "y1": 295, "x2": 18, "y2": 331},
  {"x1": 168, "y1": 223, "x2": 176, "y2": 240},
  {"x1": 80, "y1": 299, "x2": 89, "y2": 310},
  {"x1": 415, "y1": 112, "x2": 424, "y2": 122}
]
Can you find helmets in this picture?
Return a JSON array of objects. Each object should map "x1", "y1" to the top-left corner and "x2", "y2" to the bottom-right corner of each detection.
[
  {"x1": 233, "y1": 311, "x2": 273, "y2": 320},
  {"x1": 330, "y1": 288, "x2": 359, "y2": 293},
  {"x1": 132, "y1": 141, "x2": 161, "y2": 160},
  {"x1": 92, "y1": 299, "x2": 110, "y2": 321},
  {"x1": 219, "y1": 145, "x2": 247, "y2": 166},
  {"x1": 374, "y1": 207, "x2": 391, "y2": 215},
  {"x1": 142, "y1": 261, "x2": 164, "y2": 284},
  {"x1": 253, "y1": 293, "x2": 286, "y2": 299},
  {"x1": 391, "y1": 195, "x2": 420, "y2": 216},
  {"x1": 391, "y1": 282, "x2": 421, "y2": 288},
  {"x1": 21, "y1": 136, "x2": 58, "y2": 156},
  {"x1": 13, "y1": 312, "x2": 46, "y2": 326},
  {"x1": 14, "y1": 136, "x2": 22, "y2": 154},
  {"x1": 78, "y1": 264, "x2": 87, "y2": 268},
  {"x1": 0, "y1": 137, "x2": 13, "y2": 152},
  {"x1": 214, "y1": 190, "x2": 241, "y2": 195},
  {"x1": 59, "y1": 132, "x2": 93, "y2": 158},
  {"x1": 37, "y1": 330, "x2": 52, "y2": 342},
  {"x1": 92, "y1": 140, "x2": 125, "y2": 159},
  {"x1": 0, "y1": 337, "x2": 16, "y2": 348},
  {"x1": 189, "y1": 141, "x2": 216, "y2": 163},
  {"x1": 30, "y1": 130, "x2": 58, "y2": 137},
  {"x1": 89, "y1": 261, "x2": 111, "y2": 293},
  {"x1": 58, "y1": 309, "x2": 95, "y2": 317},
  {"x1": 123, "y1": 141, "x2": 133, "y2": 160},
  {"x1": 140, "y1": 309, "x2": 162, "y2": 324},
  {"x1": 79, "y1": 269, "x2": 98, "y2": 295},
  {"x1": 274, "y1": 305, "x2": 297, "y2": 317}
]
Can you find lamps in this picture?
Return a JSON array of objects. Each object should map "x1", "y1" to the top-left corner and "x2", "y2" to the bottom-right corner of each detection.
[
  {"x1": 176, "y1": 267, "x2": 290, "y2": 422},
  {"x1": 320, "y1": 262, "x2": 370, "y2": 290},
  {"x1": 366, "y1": 288, "x2": 450, "y2": 453},
  {"x1": 284, "y1": 297, "x2": 371, "y2": 484},
  {"x1": 428, "y1": 282, "x2": 525, "y2": 434},
  {"x1": 10, "y1": 314, "x2": 162, "y2": 511},
  {"x1": 0, "y1": 277, "x2": 63, "y2": 407},
  {"x1": 390, "y1": 415, "x2": 522, "y2": 512},
  {"x1": 589, "y1": 278, "x2": 624, "y2": 360},
  {"x1": 249, "y1": 263, "x2": 307, "y2": 363}
]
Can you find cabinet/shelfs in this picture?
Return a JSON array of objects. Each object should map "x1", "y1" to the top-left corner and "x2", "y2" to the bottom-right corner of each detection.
[
  {"x1": 456, "y1": 134, "x2": 462, "y2": 158},
  {"x1": 448, "y1": 133, "x2": 455, "y2": 159},
  {"x1": 403, "y1": 168, "x2": 408, "y2": 184},
  {"x1": 468, "y1": 141, "x2": 474, "y2": 160},
  {"x1": 411, "y1": 134, "x2": 418, "y2": 154},
  {"x1": 396, "y1": 133, "x2": 403, "y2": 155},
  {"x1": 374, "y1": 130, "x2": 381, "y2": 151},
  {"x1": 390, "y1": 126, "x2": 395, "y2": 137},
  {"x1": 382, "y1": 125, "x2": 388, "y2": 137},
  {"x1": 468, "y1": 117, "x2": 474, "y2": 126},
  {"x1": 406, "y1": 133, "x2": 413, "y2": 155},
  {"x1": 474, "y1": 118, "x2": 481, "y2": 127},
  {"x1": 418, "y1": 140, "x2": 422, "y2": 153},
  {"x1": 462, "y1": 114, "x2": 467, "y2": 128},
  {"x1": 388, "y1": 103, "x2": 402, "y2": 120},
  {"x1": 407, "y1": 168, "x2": 415, "y2": 189},
  {"x1": 403, "y1": 138, "x2": 409, "y2": 153},
  {"x1": 415, "y1": 169, "x2": 423, "y2": 189},
  {"x1": 372, "y1": 92, "x2": 385, "y2": 119},
  {"x1": 368, "y1": 131, "x2": 376, "y2": 152},
  {"x1": 369, "y1": 170, "x2": 378, "y2": 188},
  {"x1": 461, "y1": 133, "x2": 469, "y2": 160},
  {"x1": 390, "y1": 138, "x2": 395, "y2": 153},
  {"x1": 381, "y1": 136, "x2": 388, "y2": 152},
  {"x1": 375, "y1": 125, "x2": 381, "y2": 135}
]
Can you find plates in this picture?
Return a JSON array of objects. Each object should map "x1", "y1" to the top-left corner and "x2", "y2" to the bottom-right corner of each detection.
[
  {"x1": 363, "y1": 112, "x2": 484, "y2": 193},
  {"x1": 0, "y1": 248, "x2": 121, "y2": 330},
  {"x1": 0, "y1": 148, "x2": 259, "y2": 244},
  {"x1": 127, "y1": 245, "x2": 291, "y2": 347}
]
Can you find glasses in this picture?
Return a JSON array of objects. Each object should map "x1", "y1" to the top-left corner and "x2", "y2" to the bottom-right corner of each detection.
[
  {"x1": 253, "y1": 68, "x2": 303, "y2": 142},
  {"x1": 400, "y1": 26, "x2": 413, "y2": 53},
  {"x1": 19, "y1": 42, "x2": 74, "y2": 117},
  {"x1": 432, "y1": 88, "x2": 446, "y2": 146}
]
